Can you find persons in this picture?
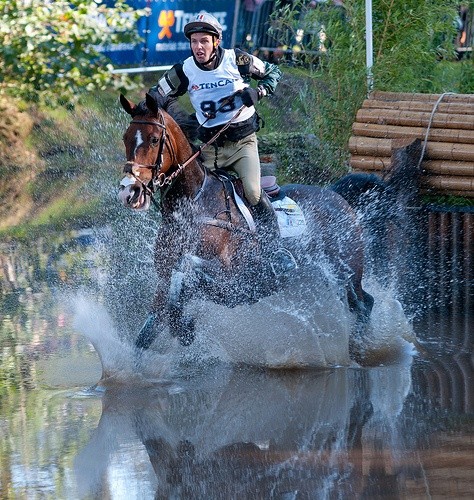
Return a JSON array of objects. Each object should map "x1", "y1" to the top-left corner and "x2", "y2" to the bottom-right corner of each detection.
[{"x1": 138, "y1": 13, "x2": 284, "y2": 271}]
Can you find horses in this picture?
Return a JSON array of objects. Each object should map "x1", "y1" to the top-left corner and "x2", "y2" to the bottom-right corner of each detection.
[{"x1": 114, "y1": 92, "x2": 410, "y2": 369}]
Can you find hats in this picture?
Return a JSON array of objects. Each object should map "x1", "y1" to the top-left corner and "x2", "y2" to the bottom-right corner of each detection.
[{"x1": 184, "y1": 12, "x2": 223, "y2": 41}]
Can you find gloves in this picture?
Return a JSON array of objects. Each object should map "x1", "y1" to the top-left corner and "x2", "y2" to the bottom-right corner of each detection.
[{"x1": 240, "y1": 87, "x2": 258, "y2": 108}]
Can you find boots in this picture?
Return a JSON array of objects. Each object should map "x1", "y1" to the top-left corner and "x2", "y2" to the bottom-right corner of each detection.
[{"x1": 250, "y1": 188, "x2": 280, "y2": 245}]
